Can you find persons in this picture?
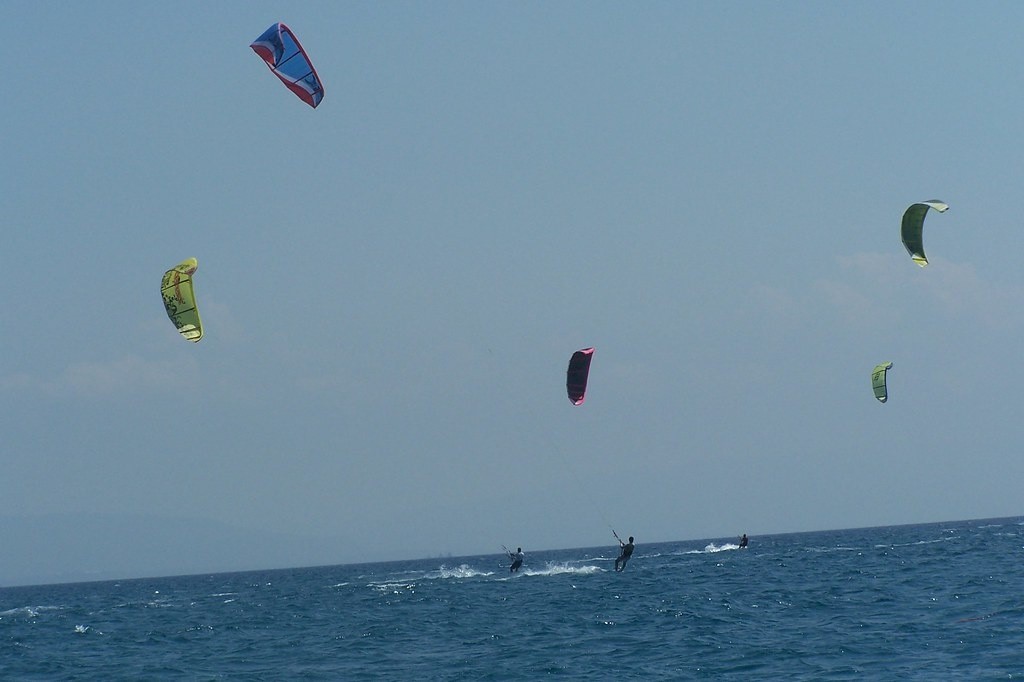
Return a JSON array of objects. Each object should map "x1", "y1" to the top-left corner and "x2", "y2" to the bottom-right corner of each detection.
[
  {"x1": 614, "y1": 536, "x2": 635, "y2": 573},
  {"x1": 739, "y1": 534, "x2": 749, "y2": 549},
  {"x1": 510, "y1": 547, "x2": 524, "y2": 573}
]
